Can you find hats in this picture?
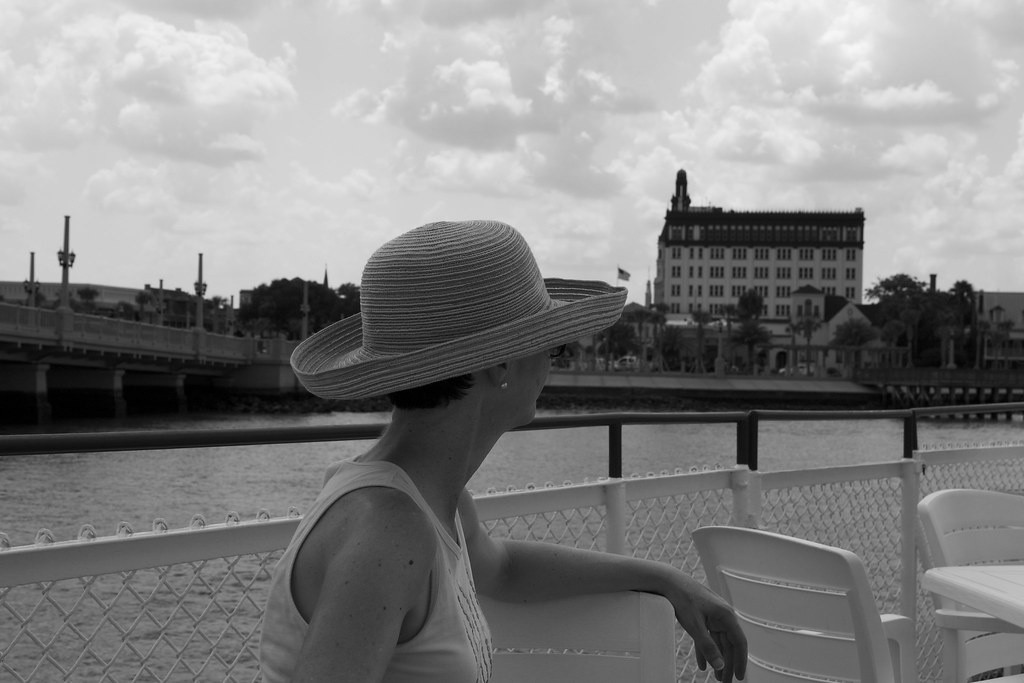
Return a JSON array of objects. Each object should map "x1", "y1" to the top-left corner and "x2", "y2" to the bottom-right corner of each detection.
[{"x1": 288, "y1": 220, "x2": 627, "y2": 401}]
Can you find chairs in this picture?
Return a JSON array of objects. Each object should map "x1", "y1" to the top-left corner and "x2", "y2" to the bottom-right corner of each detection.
[
  {"x1": 691, "y1": 525, "x2": 916, "y2": 683},
  {"x1": 475, "y1": 585, "x2": 681, "y2": 683},
  {"x1": 918, "y1": 487, "x2": 1024, "y2": 683}
]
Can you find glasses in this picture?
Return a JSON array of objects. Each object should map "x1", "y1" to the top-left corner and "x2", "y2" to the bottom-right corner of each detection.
[{"x1": 550, "y1": 345, "x2": 566, "y2": 358}]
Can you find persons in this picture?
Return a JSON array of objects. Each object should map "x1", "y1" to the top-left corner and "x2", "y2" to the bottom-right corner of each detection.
[{"x1": 258, "y1": 220, "x2": 752, "y2": 683}]
